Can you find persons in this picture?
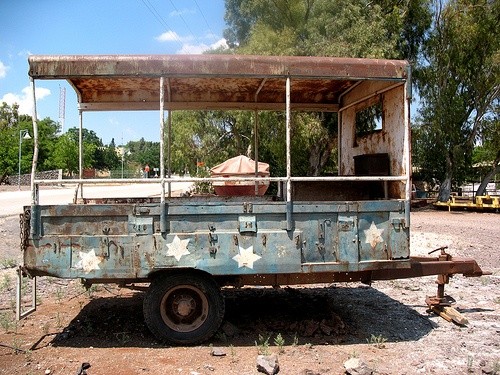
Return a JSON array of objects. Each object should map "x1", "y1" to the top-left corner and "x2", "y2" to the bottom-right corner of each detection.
[{"x1": 144, "y1": 163, "x2": 149, "y2": 178}]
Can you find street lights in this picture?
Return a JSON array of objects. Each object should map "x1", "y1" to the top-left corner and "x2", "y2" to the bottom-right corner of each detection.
[{"x1": 17, "y1": 129, "x2": 31, "y2": 188}]
